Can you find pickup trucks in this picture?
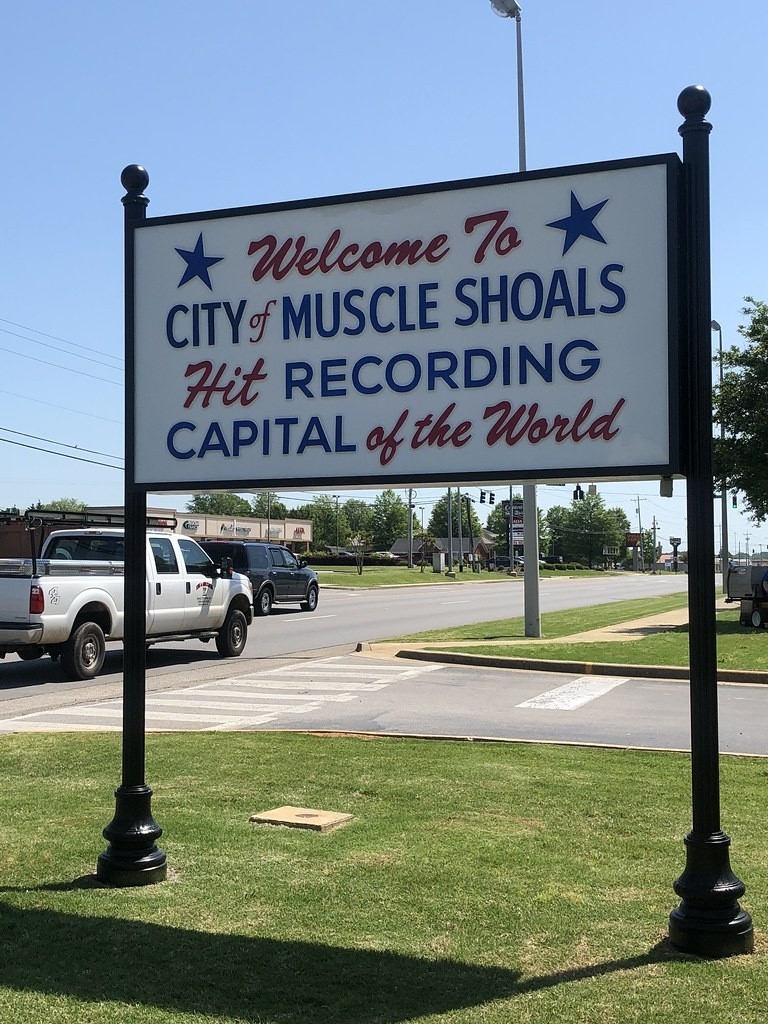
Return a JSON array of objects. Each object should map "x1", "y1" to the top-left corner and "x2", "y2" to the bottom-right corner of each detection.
[{"x1": 0, "y1": 527, "x2": 254, "y2": 681}]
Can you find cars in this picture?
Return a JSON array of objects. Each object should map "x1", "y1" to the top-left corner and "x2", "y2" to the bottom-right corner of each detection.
[
  {"x1": 517, "y1": 556, "x2": 546, "y2": 564},
  {"x1": 486, "y1": 556, "x2": 524, "y2": 568}
]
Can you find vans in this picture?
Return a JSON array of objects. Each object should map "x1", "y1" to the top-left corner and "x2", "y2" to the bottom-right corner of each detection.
[{"x1": 540, "y1": 556, "x2": 564, "y2": 564}]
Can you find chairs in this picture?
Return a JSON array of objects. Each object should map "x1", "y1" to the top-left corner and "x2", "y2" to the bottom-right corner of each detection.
[{"x1": 58, "y1": 543, "x2": 167, "y2": 571}]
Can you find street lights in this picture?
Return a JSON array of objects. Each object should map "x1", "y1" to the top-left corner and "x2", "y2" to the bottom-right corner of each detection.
[
  {"x1": 710, "y1": 319, "x2": 734, "y2": 604},
  {"x1": 489, "y1": 1, "x2": 540, "y2": 636}
]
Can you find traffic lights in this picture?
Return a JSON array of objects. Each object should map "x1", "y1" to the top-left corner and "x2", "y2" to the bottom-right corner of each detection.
[
  {"x1": 490, "y1": 493, "x2": 495, "y2": 504},
  {"x1": 733, "y1": 496, "x2": 737, "y2": 508},
  {"x1": 480, "y1": 491, "x2": 486, "y2": 503}
]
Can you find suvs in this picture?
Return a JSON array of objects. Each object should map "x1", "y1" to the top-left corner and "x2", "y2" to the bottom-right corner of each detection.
[{"x1": 186, "y1": 538, "x2": 320, "y2": 617}]
